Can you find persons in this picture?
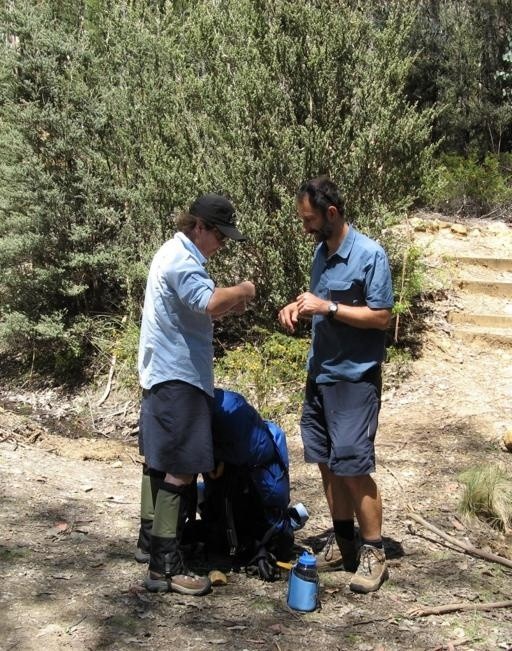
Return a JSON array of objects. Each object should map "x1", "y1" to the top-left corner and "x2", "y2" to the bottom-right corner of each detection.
[
  {"x1": 128, "y1": 192, "x2": 278, "y2": 601},
  {"x1": 262, "y1": 175, "x2": 403, "y2": 595}
]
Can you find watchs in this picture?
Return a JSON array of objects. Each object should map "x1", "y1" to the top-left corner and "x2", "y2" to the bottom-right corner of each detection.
[{"x1": 326, "y1": 299, "x2": 340, "y2": 318}]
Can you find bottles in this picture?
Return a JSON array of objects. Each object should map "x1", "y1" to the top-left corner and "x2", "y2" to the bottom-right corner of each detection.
[{"x1": 287, "y1": 551, "x2": 318, "y2": 612}]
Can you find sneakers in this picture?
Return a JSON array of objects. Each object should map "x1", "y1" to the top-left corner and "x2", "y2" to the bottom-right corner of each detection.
[
  {"x1": 134, "y1": 542, "x2": 212, "y2": 596},
  {"x1": 314, "y1": 534, "x2": 359, "y2": 571},
  {"x1": 349, "y1": 543, "x2": 389, "y2": 593}
]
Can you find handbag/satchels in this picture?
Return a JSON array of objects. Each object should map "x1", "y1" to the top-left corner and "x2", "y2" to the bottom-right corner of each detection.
[{"x1": 206, "y1": 379, "x2": 310, "y2": 581}]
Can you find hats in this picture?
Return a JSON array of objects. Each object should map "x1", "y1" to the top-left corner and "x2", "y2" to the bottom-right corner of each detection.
[{"x1": 187, "y1": 193, "x2": 247, "y2": 243}]
